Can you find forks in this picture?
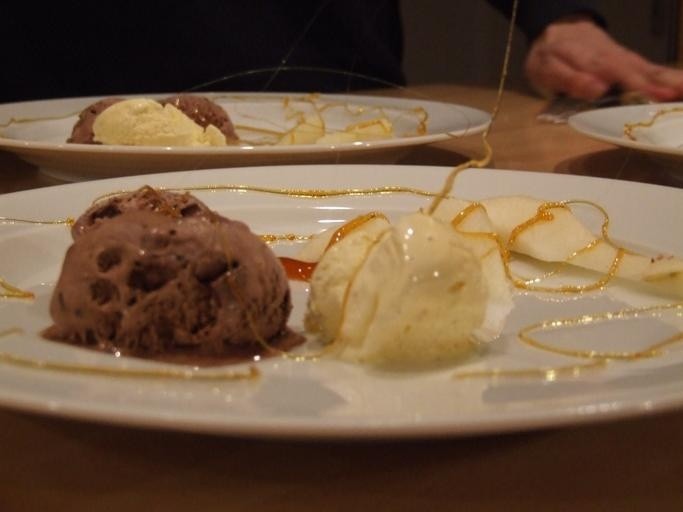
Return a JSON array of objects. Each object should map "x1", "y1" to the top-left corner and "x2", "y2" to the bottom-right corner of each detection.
[{"x1": 564, "y1": 101, "x2": 682, "y2": 164}]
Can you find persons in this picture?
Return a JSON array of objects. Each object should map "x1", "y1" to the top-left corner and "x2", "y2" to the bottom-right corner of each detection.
[{"x1": 1, "y1": 0, "x2": 683, "y2": 104}]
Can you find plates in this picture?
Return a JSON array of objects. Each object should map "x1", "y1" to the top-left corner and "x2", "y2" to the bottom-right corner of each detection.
[
  {"x1": 0, "y1": 161, "x2": 682, "y2": 437},
  {"x1": 1, "y1": 90, "x2": 493, "y2": 184}
]
